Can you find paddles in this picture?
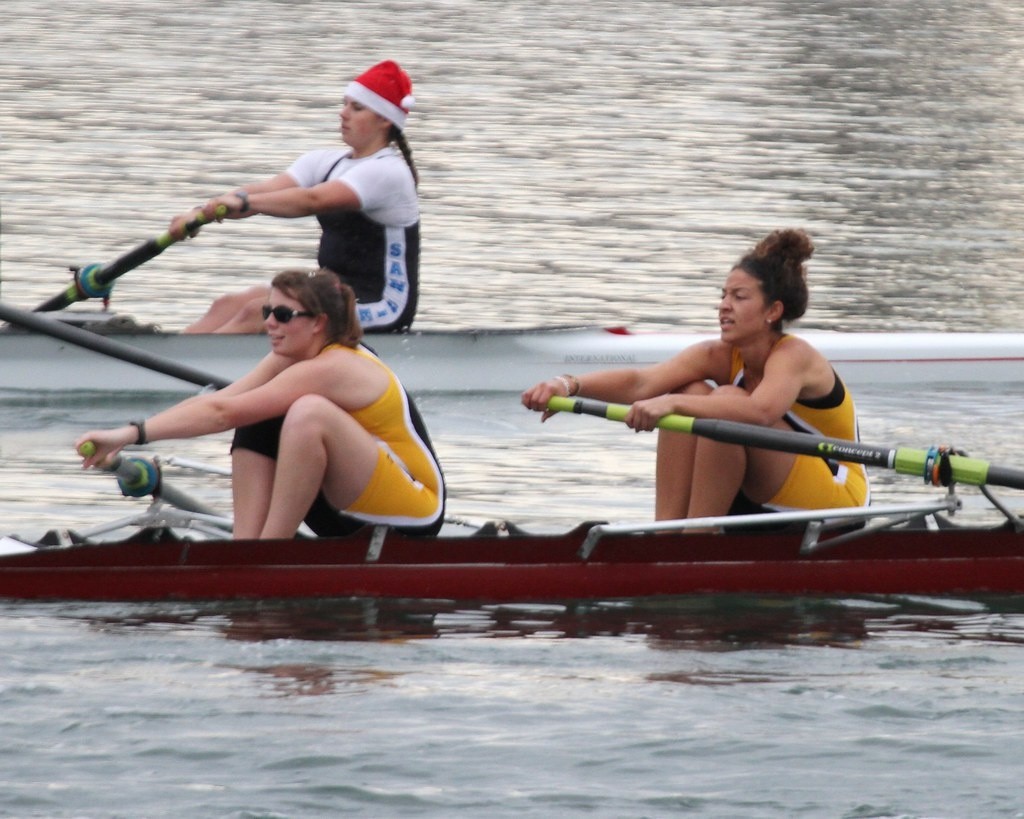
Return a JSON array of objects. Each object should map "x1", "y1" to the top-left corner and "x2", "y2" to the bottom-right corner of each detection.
[
  {"x1": 31, "y1": 203, "x2": 227, "y2": 314},
  {"x1": 547, "y1": 396, "x2": 1024, "y2": 494},
  {"x1": 78, "y1": 440, "x2": 233, "y2": 541}
]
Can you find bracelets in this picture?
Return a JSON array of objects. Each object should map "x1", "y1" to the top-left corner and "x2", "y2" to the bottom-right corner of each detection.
[
  {"x1": 555, "y1": 374, "x2": 580, "y2": 397},
  {"x1": 193, "y1": 204, "x2": 204, "y2": 209}
]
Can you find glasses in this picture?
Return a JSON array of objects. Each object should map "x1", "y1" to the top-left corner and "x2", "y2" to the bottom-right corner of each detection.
[{"x1": 262, "y1": 305, "x2": 314, "y2": 323}]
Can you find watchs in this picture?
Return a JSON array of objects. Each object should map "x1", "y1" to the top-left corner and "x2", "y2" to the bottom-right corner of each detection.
[
  {"x1": 235, "y1": 192, "x2": 249, "y2": 215},
  {"x1": 130, "y1": 418, "x2": 146, "y2": 445}
]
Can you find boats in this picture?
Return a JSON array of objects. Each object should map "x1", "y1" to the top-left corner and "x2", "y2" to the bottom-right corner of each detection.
[
  {"x1": 1, "y1": 328, "x2": 1024, "y2": 397},
  {"x1": 1, "y1": 517, "x2": 1024, "y2": 599}
]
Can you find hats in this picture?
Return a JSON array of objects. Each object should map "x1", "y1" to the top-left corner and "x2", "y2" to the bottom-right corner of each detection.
[{"x1": 346, "y1": 60, "x2": 415, "y2": 129}]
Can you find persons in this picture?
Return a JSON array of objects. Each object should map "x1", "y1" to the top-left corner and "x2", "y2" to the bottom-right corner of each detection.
[
  {"x1": 522, "y1": 229, "x2": 871, "y2": 523},
  {"x1": 168, "y1": 59, "x2": 421, "y2": 334},
  {"x1": 75, "y1": 267, "x2": 445, "y2": 538}
]
